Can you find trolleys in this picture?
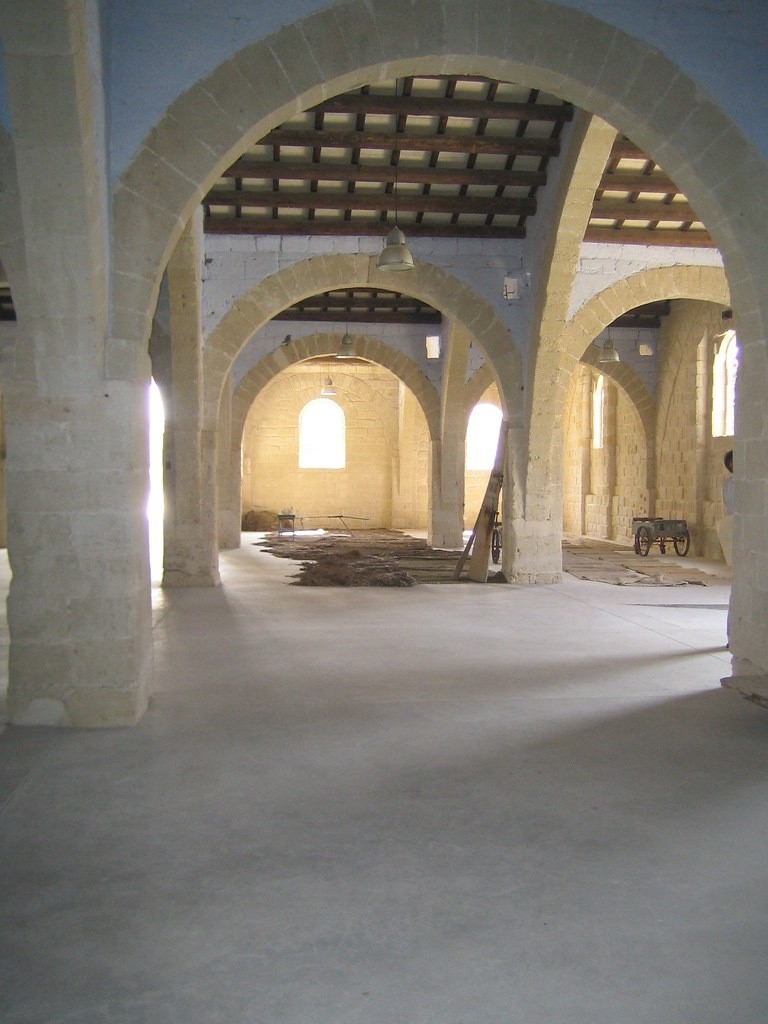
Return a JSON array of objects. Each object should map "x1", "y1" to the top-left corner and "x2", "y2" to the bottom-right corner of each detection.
[{"x1": 630, "y1": 516, "x2": 690, "y2": 557}]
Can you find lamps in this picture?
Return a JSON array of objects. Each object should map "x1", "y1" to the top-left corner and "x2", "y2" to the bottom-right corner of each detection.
[
  {"x1": 597, "y1": 326, "x2": 620, "y2": 364},
  {"x1": 425, "y1": 335, "x2": 442, "y2": 359},
  {"x1": 341, "y1": 320, "x2": 353, "y2": 345},
  {"x1": 375, "y1": 79, "x2": 416, "y2": 272}
]
